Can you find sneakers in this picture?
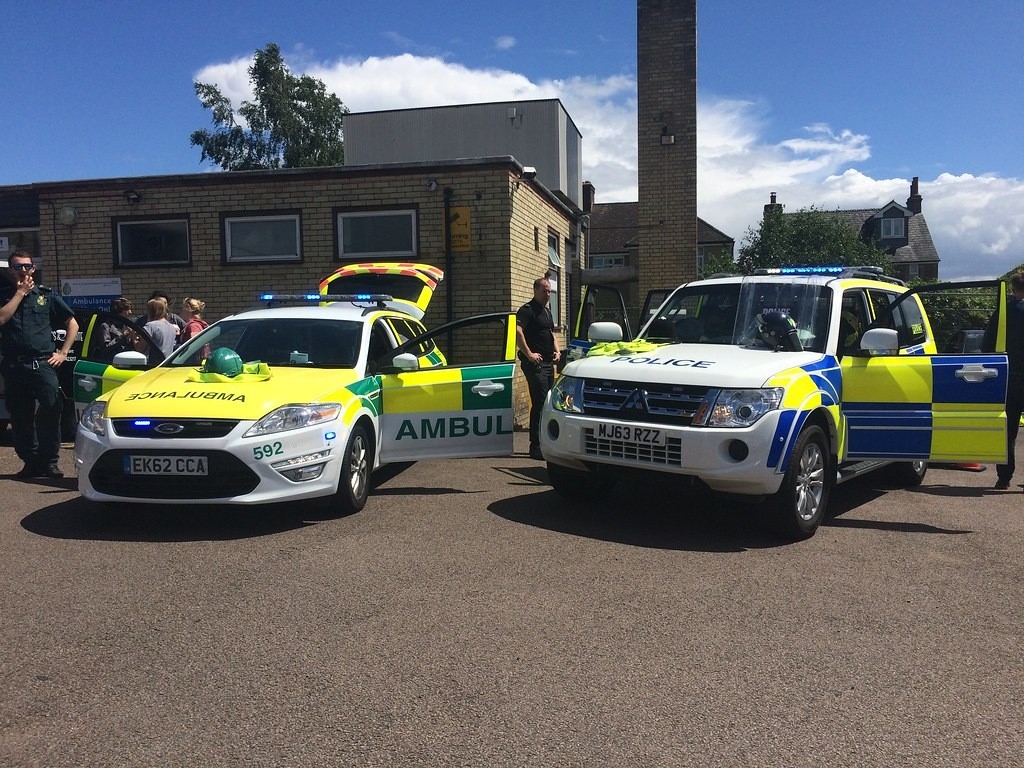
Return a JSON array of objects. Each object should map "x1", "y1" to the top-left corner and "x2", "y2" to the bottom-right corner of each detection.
[
  {"x1": 41, "y1": 463, "x2": 64, "y2": 479},
  {"x1": 16, "y1": 461, "x2": 47, "y2": 478}
]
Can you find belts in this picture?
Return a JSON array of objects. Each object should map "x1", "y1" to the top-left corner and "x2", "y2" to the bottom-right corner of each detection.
[{"x1": 4, "y1": 351, "x2": 53, "y2": 363}]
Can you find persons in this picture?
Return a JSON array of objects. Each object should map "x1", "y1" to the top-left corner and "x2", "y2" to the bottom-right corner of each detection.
[
  {"x1": 516, "y1": 278, "x2": 562, "y2": 461},
  {"x1": 101, "y1": 290, "x2": 211, "y2": 366},
  {"x1": 0, "y1": 249, "x2": 79, "y2": 478},
  {"x1": 979, "y1": 270, "x2": 1024, "y2": 493}
]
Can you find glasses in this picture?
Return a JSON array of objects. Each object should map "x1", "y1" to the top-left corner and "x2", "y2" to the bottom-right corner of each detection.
[{"x1": 9, "y1": 263, "x2": 33, "y2": 271}]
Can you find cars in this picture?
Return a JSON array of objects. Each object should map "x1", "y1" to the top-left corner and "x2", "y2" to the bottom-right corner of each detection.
[{"x1": 941, "y1": 329, "x2": 985, "y2": 355}]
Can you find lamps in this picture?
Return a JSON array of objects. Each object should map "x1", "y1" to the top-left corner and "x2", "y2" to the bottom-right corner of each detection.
[
  {"x1": 123, "y1": 189, "x2": 142, "y2": 204},
  {"x1": 505, "y1": 107, "x2": 518, "y2": 127},
  {"x1": 659, "y1": 135, "x2": 678, "y2": 146},
  {"x1": 514, "y1": 166, "x2": 539, "y2": 189},
  {"x1": 425, "y1": 178, "x2": 438, "y2": 193},
  {"x1": 59, "y1": 205, "x2": 79, "y2": 227}
]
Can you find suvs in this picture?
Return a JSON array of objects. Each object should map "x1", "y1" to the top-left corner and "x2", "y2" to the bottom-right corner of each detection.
[{"x1": 537, "y1": 263, "x2": 1012, "y2": 542}]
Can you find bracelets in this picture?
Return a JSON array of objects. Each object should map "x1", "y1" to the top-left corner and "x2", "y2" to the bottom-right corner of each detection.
[{"x1": 558, "y1": 352, "x2": 562, "y2": 355}]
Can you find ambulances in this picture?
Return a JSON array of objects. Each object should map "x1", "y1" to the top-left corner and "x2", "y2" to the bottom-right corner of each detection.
[{"x1": 71, "y1": 262, "x2": 521, "y2": 511}]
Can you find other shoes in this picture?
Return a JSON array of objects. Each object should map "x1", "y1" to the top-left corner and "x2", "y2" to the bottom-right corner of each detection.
[
  {"x1": 530, "y1": 448, "x2": 546, "y2": 461},
  {"x1": 995, "y1": 479, "x2": 1010, "y2": 489}
]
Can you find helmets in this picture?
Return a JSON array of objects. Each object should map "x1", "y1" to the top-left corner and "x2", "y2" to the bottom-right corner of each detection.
[
  {"x1": 756, "y1": 311, "x2": 796, "y2": 351},
  {"x1": 205, "y1": 348, "x2": 243, "y2": 378}
]
